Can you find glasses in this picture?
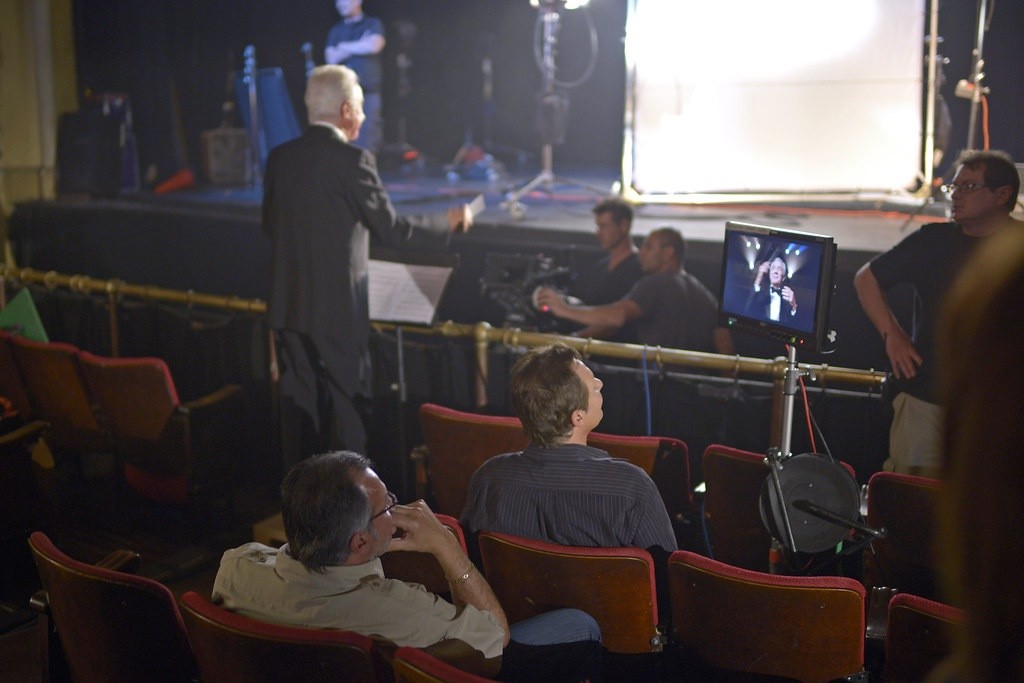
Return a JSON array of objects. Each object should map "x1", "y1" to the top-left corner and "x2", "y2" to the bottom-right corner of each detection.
[
  {"x1": 370, "y1": 491, "x2": 398, "y2": 522},
  {"x1": 945, "y1": 183, "x2": 989, "y2": 193}
]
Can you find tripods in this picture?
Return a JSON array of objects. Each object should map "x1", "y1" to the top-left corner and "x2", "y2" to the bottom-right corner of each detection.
[{"x1": 499, "y1": 0, "x2": 614, "y2": 211}]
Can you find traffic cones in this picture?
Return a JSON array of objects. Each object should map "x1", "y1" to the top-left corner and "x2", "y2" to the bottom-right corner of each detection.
[{"x1": 154, "y1": 166, "x2": 195, "y2": 196}]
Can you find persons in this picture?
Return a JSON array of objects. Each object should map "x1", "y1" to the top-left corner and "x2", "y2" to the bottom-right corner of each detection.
[
  {"x1": 580, "y1": 198, "x2": 643, "y2": 366},
  {"x1": 538, "y1": 228, "x2": 735, "y2": 380},
  {"x1": 932, "y1": 229, "x2": 1024, "y2": 683},
  {"x1": 207, "y1": 449, "x2": 602, "y2": 682},
  {"x1": 855, "y1": 149, "x2": 1024, "y2": 478},
  {"x1": 745, "y1": 257, "x2": 802, "y2": 329},
  {"x1": 263, "y1": 64, "x2": 471, "y2": 464},
  {"x1": 456, "y1": 342, "x2": 680, "y2": 627},
  {"x1": 323, "y1": 0, "x2": 386, "y2": 153}
]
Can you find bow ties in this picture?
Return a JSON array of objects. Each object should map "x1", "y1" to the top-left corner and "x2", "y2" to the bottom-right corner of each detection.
[{"x1": 771, "y1": 286, "x2": 781, "y2": 296}]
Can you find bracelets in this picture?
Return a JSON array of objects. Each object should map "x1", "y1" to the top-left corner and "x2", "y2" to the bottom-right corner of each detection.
[{"x1": 444, "y1": 562, "x2": 475, "y2": 584}]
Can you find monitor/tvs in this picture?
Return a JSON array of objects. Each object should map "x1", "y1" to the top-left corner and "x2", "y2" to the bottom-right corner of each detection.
[{"x1": 717, "y1": 220, "x2": 838, "y2": 352}]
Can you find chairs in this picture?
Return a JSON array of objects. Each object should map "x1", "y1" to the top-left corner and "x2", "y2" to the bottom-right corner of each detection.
[{"x1": 0, "y1": 330, "x2": 979, "y2": 683}]
